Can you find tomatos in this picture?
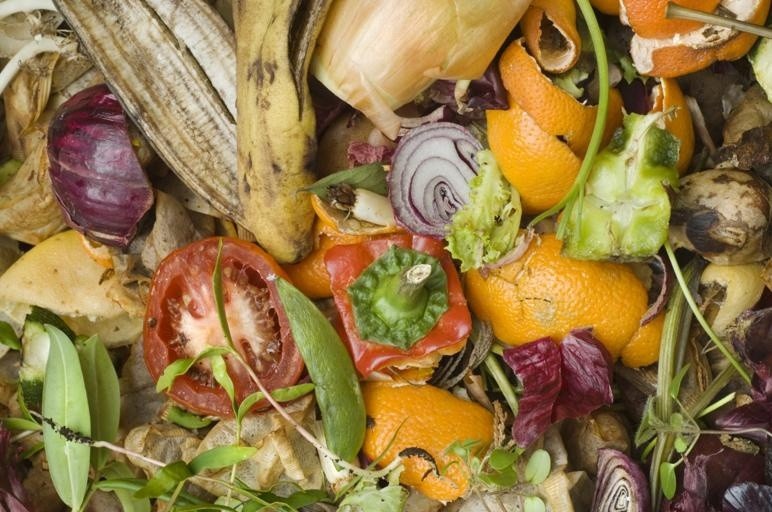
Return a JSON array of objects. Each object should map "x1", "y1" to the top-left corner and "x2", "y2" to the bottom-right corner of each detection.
[{"x1": 144, "y1": 237, "x2": 304, "y2": 417}]
